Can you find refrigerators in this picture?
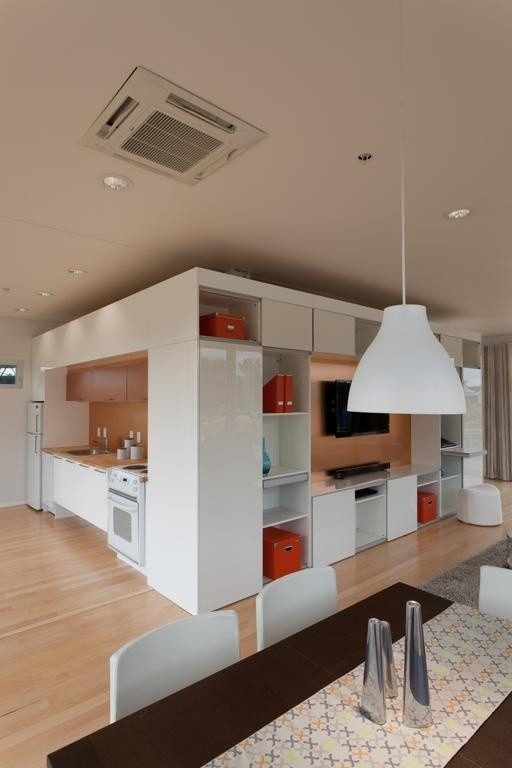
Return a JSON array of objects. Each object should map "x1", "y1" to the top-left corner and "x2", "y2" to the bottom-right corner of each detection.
[{"x1": 25, "y1": 400, "x2": 44, "y2": 513}]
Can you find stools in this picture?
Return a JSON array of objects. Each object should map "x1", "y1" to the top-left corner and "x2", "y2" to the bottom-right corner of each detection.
[{"x1": 455, "y1": 482, "x2": 505, "y2": 527}]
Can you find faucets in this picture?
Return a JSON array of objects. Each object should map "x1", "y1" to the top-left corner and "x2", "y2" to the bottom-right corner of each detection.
[{"x1": 90, "y1": 438, "x2": 111, "y2": 453}]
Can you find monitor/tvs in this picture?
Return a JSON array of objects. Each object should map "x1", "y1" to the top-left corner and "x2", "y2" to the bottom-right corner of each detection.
[{"x1": 335, "y1": 380, "x2": 390, "y2": 438}]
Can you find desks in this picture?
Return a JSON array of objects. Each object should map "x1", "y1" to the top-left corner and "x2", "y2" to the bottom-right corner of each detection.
[{"x1": 42, "y1": 579, "x2": 511, "y2": 767}]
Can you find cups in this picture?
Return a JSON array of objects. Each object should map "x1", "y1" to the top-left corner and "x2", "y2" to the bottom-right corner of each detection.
[
  {"x1": 129, "y1": 445, "x2": 143, "y2": 459},
  {"x1": 122, "y1": 437, "x2": 135, "y2": 449},
  {"x1": 115, "y1": 447, "x2": 128, "y2": 460}
]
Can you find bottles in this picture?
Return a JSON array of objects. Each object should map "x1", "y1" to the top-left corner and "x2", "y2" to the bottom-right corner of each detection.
[
  {"x1": 135, "y1": 431, "x2": 143, "y2": 446},
  {"x1": 128, "y1": 430, "x2": 134, "y2": 439}
]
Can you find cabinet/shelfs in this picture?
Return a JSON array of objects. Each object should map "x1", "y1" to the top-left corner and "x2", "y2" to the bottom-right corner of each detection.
[
  {"x1": 125, "y1": 356, "x2": 148, "y2": 402},
  {"x1": 259, "y1": 295, "x2": 358, "y2": 357},
  {"x1": 260, "y1": 346, "x2": 312, "y2": 578},
  {"x1": 307, "y1": 473, "x2": 418, "y2": 568},
  {"x1": 411, "y1": 331, "x2": 490, "y2": 529},
  {"x1": 51, "y1": 453, "x2": 108, "y2": 533},
  {"x1": 63, "y1": 364, "x2": 129, "y2": 404},
  {"x1": 39, "y1": 449, "x2": 77, "y2": 519}
]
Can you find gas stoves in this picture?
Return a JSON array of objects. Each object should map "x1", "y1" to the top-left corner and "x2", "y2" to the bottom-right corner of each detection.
[{"x1": 106, "y1": 461, "x2": 148, "y2": 500}]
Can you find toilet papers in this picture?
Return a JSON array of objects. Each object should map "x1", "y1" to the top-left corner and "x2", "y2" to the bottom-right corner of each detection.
[
  {"x1": 117, "y1": 448, "x2": 127, "y2": 460},
  {"x1": 124, "y1": 439, "x2": 132, "y2": 459},
  {"x1": 130, "y1": 446, "x2": 142, "y2": 460}
]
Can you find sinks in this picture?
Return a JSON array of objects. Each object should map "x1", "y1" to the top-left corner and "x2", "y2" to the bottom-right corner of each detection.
[{"x1": 67, "y1": 447, "x2": 108, "y2": 455}]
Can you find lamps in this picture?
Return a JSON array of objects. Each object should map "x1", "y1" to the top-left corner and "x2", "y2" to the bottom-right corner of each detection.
[{"x1": 345, "y1": 126, "x2": 470, "y2": 415}]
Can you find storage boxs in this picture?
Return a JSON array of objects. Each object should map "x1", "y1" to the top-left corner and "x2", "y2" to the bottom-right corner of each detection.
[
  {"x1": 416, "y1": 488, "x2": 440, "y2": 525},
  {"x1": 197, "y1": 311, "x2": 249, "y2": 340},
  {"x1": 262, "y1": 525, "x2": 303, "y2": 578}
]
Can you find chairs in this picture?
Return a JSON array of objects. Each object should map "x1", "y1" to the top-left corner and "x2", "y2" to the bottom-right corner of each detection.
[
  {"x1": 254, "y1": 564, "x2": 340, "y2": 655},
  {"x1": 107, "y1": 607, "x2": 243, "y2": 723},
  {"x1": 478, "y1": 564, "x2": 512, "y2": 619}
]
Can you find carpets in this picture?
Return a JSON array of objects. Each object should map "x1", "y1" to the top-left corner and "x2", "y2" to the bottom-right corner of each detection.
[{"x1": 417, "y1": 536, "x2": 512, "y2": 610}]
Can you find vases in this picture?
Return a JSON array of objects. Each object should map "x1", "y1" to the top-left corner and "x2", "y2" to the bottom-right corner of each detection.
[
  {"x1": 262, "y1": 437, "x2": 272, "y2": 476},
  {"x1": 358, "y1": 597, "x2": 433, "y2": 729}
]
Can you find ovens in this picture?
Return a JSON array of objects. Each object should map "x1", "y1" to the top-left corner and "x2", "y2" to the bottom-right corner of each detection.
[{"x1": 103, "y1": 486, "x2": 143, "y2": 569}]
